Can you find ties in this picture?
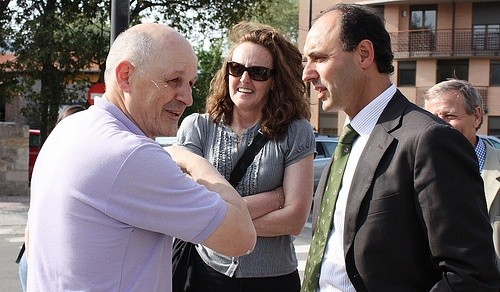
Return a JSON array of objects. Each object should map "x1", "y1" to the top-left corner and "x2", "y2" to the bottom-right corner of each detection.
[{"x1": 300, "y1": 124, "x2": 359, "y2": 292}]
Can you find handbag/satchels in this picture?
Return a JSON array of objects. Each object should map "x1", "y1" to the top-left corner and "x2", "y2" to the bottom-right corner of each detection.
[{"x1": 171, "y1": 238, "x2": 191, "y2": 292}]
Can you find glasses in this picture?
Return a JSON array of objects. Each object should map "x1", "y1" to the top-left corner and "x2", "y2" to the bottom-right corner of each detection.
[{"x1": 226, "y1": 61, "x2": 276, "y2": 81}]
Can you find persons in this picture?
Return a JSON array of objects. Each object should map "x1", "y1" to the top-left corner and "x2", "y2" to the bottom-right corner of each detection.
[
  {"x1": 423, "y1": 78, "x2": 500, "y2": 271},
  {"x1": 26, "y1": 23, "x2": 257, "y2": 292},
  {"x1": 15, "y1": 105, "x2": 86, "y2": 292},
  {"x1": 302, "y1": 4, "x2": 500, "y2": 292},
  {"x1": 173, "y1": 21, "x2": 318, "y2": 292}
]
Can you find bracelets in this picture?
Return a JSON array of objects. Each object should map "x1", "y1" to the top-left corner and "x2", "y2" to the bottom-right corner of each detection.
[{"x1": 274, "y1": 190, "x2": 282, "y2": 208}]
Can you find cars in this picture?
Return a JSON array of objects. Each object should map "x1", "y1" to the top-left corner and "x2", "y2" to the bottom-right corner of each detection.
[{"x1": 313, "y1": 138, "x2": 339, "y2": 190}]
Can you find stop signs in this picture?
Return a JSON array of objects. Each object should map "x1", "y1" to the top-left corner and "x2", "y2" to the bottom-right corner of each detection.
[{"x1": 88, "y1": 84, "x2": 106, "y2": 105}]
